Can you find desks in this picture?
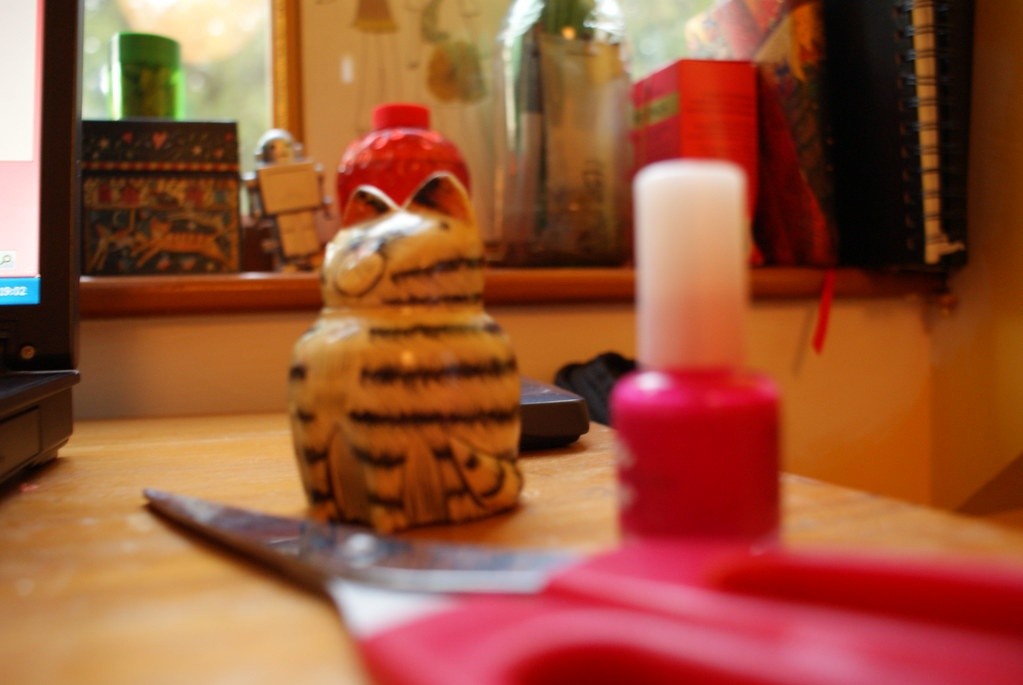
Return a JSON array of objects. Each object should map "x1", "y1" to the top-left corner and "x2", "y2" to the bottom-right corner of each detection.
[{"x1": 0, "y1": 411, "x2": 1023, "y2": 685}]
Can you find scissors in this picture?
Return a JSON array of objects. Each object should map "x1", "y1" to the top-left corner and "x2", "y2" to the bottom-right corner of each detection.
[{"x1": 137, "y1": 484, "x2": 1023, "y2": 685}]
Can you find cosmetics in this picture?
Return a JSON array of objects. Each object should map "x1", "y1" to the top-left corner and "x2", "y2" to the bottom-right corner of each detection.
[{"x1": 604, "y1": 160, "x2": 782, "y2": 543}]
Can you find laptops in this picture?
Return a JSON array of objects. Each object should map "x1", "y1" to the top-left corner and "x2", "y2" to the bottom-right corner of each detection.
[{"x1": 0, "y1": 0, "x2": 88, "y2": 485}]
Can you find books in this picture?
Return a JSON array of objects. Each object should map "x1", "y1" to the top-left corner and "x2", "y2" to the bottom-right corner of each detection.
[{"x1": 272, "y1": 0, "x2": 970, "y2": 268}]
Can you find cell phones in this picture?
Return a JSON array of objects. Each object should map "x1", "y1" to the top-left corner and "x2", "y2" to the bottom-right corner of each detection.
[{"x1": 519, "y1": 376, "x2": 590, "y2": 451}]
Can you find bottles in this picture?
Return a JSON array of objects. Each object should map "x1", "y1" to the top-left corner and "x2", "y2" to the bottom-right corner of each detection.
[
  {"x1": 336, "y1": 102, "x2": 475, "y2": 232},
  {"x1": 109, "y1": 31, "x2": 184, "y2": 122},
  {"x1": 606, "y1": 156, "x2": 786, "y2": 552}
]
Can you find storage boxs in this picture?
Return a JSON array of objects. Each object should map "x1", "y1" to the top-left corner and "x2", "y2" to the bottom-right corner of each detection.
[{"x1": 79, "y1": 120, "x2": 241, "y2": 272}]
